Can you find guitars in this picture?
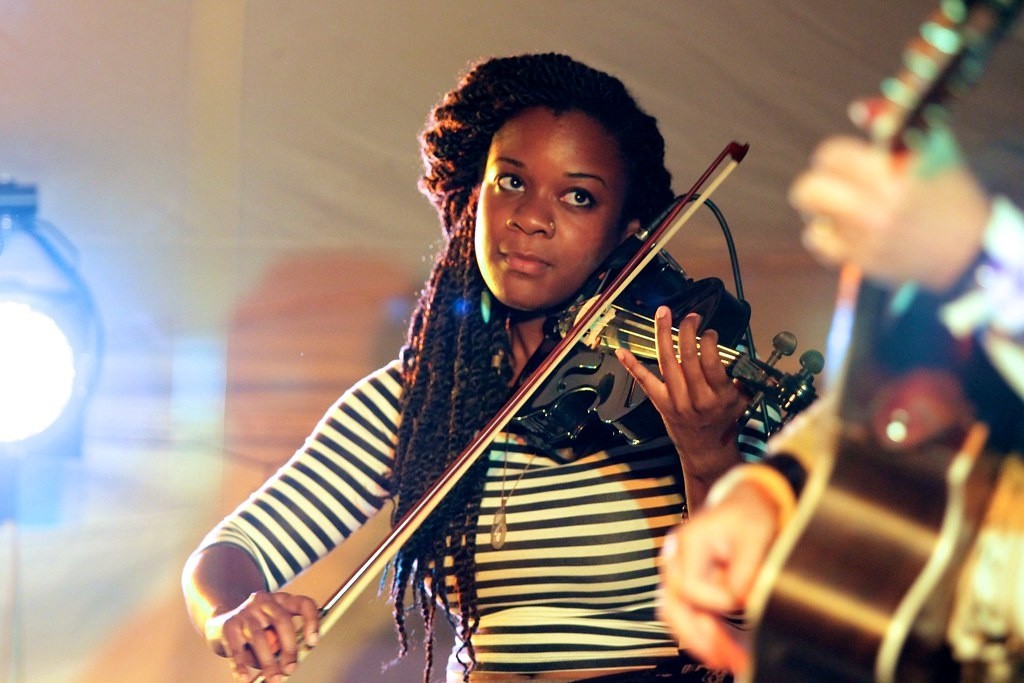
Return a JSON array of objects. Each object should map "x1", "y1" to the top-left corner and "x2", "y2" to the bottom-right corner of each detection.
[{"x1": 733, "y1": 2, "x2": 1024, "y2": 682}]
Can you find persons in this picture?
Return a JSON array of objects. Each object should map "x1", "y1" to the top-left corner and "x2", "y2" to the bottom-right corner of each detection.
[
  {"x1": 180, "y1": 52, "x2": 771, "y2": 683},
  {"x1": 654, "y1": 106, "x2": 1024, "y2": 683}
]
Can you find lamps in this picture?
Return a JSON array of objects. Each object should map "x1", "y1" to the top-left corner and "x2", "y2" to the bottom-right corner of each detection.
[{"x1": 0, "y1": 277, "x2": 93, "y2": 524}]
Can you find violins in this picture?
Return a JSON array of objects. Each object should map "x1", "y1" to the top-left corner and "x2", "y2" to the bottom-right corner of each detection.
[{"x1": 504, "y1": 233, "x2": 824, "y2": 458}]
